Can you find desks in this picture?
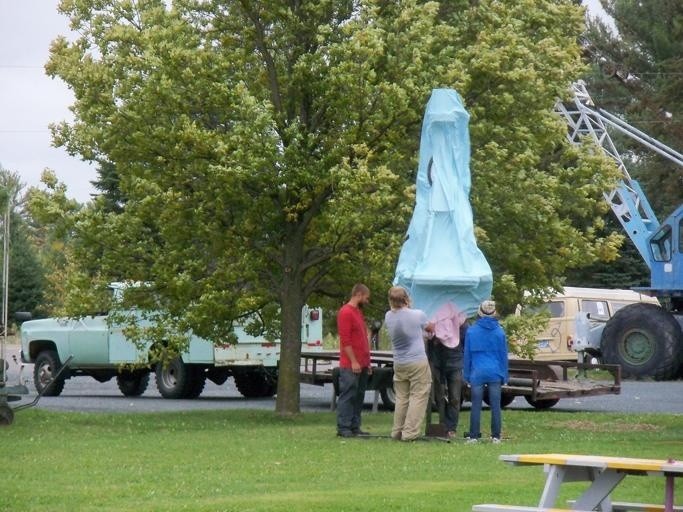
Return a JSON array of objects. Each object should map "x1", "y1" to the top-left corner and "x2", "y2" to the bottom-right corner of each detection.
[{"x1": 497, "y1": 451, "x2": 683, "y2": 512}]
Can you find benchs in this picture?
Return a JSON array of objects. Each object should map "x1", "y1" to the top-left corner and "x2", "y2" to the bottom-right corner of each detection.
[{"x1": 472, "y1": 498, "x2": 682, "y2": 512}]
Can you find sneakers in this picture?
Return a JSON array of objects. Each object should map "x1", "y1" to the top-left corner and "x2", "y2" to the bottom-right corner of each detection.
[{"x1": 336, "y1": 428, "x2": 501, "y2": 443}]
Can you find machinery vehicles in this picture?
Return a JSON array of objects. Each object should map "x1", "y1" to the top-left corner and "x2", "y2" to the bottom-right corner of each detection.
[{"x1": 549, "y1": 75, "x2": 683, "y2": 381}]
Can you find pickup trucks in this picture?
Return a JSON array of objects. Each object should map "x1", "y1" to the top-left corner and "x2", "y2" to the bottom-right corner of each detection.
[{"x1": 19, "y1": 282, "x2": 323, "y2": 399}]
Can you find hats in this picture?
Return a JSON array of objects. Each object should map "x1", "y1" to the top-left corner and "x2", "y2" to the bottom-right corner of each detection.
[{"x1": 477, "y1": 300, "x2": 497, "y2": 317}]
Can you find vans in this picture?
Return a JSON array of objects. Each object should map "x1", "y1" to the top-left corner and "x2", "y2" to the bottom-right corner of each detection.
[{"x1": 513, "y1": 288, "x2": 663, "y2": 371}]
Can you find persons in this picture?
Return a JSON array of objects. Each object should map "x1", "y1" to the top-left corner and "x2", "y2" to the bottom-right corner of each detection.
[
  {"x1": 430, "y1": 323, "x2": 465, "y2": 438},
  {"x1": 462, "y1": 301, "x2": 508, "y2": 445},
  {"x1": 385, "y1": 286, "x2": 434, "y2": 441},
  {"x1": 335, "y1": 283, "x2": 371, "y2": 438}
]
